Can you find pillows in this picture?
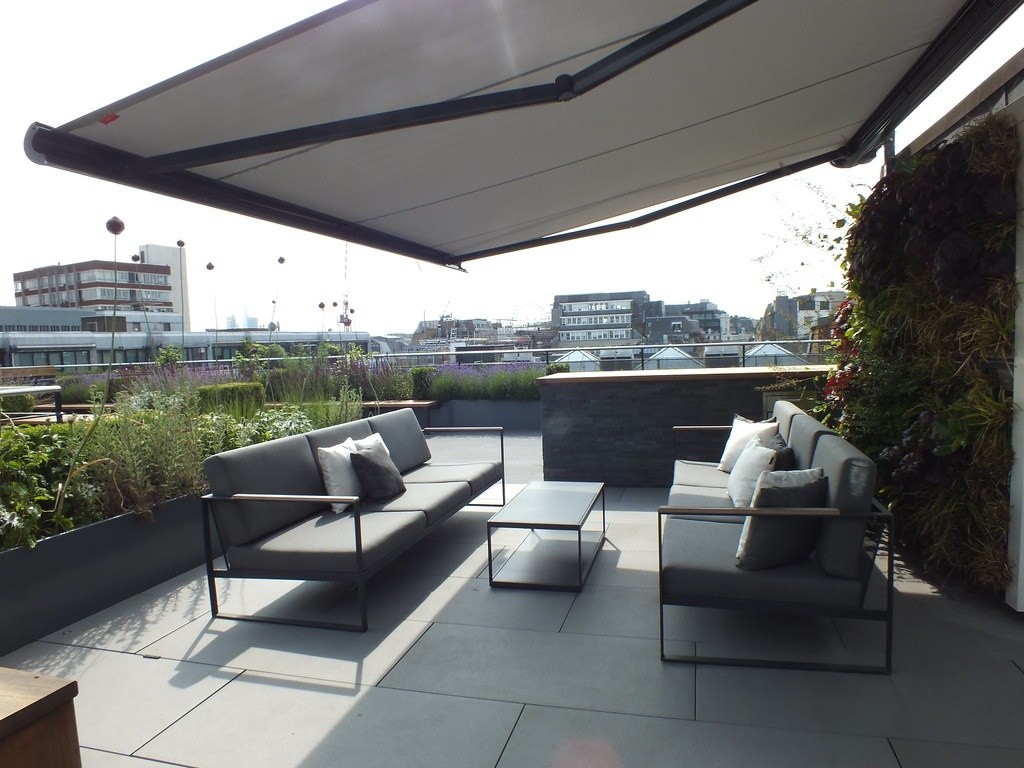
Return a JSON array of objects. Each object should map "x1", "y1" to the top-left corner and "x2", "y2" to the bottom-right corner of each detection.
[
  {"x1": 351, "y1": 440, "x2": 406, "y2": 505},
  {"x1": 735, "y1": 467, "x2": 825, "y2": 560},
  {"x1": 727, "y1": 436, "x2": 776, "y2": 506},
  {"x1": 765, "y1": 434, "x2": 796, "y2": 472},
  {"x1": 317, "y1": 438, "x2": 361, "y2": 514},
  {"x1": 731, "y1": 476, "x2": 829, "y2": 570},
  {"x1": 717, "y1": 414, "x2": 779, "y2": 474}
]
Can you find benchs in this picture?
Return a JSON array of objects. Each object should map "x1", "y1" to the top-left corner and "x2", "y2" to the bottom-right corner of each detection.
[
  {"x1": 199, "y1": 407, "x2": 504, "y2": 634},
  {"x1": 658, "y1": 400, "x2": 895, "y2": 677}
]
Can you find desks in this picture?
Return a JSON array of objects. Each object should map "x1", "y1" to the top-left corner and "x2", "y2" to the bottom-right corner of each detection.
[{"x1": 0, "y1": 667, "x2": 82, "y2": 768}]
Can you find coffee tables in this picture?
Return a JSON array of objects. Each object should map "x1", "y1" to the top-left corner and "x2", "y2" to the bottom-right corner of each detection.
[{"x1": 486, "y1": 480, "x2": 606, "y2": 592}]
